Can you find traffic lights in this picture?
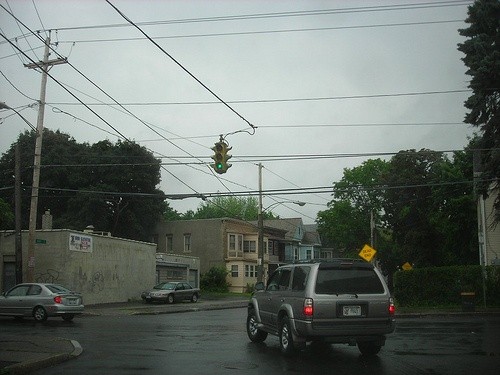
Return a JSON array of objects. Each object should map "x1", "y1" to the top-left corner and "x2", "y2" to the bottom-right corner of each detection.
[{"x1": 211, "y1": 142, "x2": 233, "y2": 173}]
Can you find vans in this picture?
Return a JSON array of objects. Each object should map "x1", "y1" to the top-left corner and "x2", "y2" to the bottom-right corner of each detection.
[{"x1": 246, "y1": 258, "x2": 396, "y2": 354}]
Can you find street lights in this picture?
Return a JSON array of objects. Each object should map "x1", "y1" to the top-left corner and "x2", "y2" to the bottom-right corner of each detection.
[
  {"x1": 0, "y1": 101, "x2": 42, "y2": 283},
  {"x1": 257, "y1": 200, "x2": 306, "y2": 285}
]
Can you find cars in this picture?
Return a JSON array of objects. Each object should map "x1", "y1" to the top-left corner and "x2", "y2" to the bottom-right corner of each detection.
[
  {"x1": 141, "y1": 280, "x2": 202, "y2": 304},
  {"x1": 0, "y1": 283, "x2": 84, "y2": 324}
]
